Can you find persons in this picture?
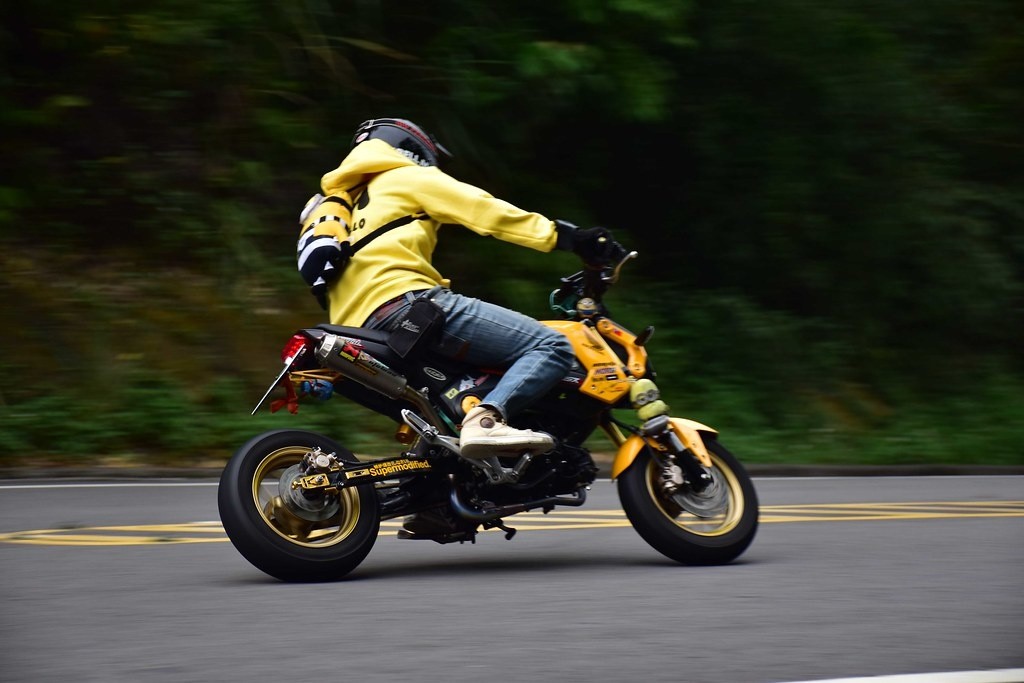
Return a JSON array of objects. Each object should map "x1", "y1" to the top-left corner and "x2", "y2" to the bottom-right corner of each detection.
[{"x1": 321, "y1": 119, "x2": 628, "y2": 544}]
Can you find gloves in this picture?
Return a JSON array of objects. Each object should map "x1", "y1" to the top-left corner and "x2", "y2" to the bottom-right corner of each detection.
[{"x1": 553, "y1": 219, "x2": 627, "y2": 265}]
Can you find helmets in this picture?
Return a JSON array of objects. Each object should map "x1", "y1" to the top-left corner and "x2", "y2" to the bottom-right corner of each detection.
[{"x1": 349, "y1": 118, "x2": 452, "y2": 167}]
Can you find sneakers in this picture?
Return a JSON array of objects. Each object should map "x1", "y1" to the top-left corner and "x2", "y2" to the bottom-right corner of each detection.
[
  {"x1": 459, "y1": 407, "x2": 554, "y2": 459},
  {"x1": 402, "y1": 512, "x2": 450, "y2": 533}
]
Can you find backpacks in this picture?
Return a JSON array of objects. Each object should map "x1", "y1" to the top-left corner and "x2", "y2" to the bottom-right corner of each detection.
[{"x1": 298, "y1": 180, "x2": 431, "y2": 311}]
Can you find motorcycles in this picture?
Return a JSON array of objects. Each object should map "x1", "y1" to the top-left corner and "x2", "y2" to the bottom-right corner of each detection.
[{"x1": 217, "y1": 233, "x2": 761, "y2": 582}]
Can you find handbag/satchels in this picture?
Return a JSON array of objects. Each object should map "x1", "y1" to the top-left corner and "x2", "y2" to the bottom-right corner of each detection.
[{"x1": 387, "y1": 298, "x2": 446, "y2": 362}]
